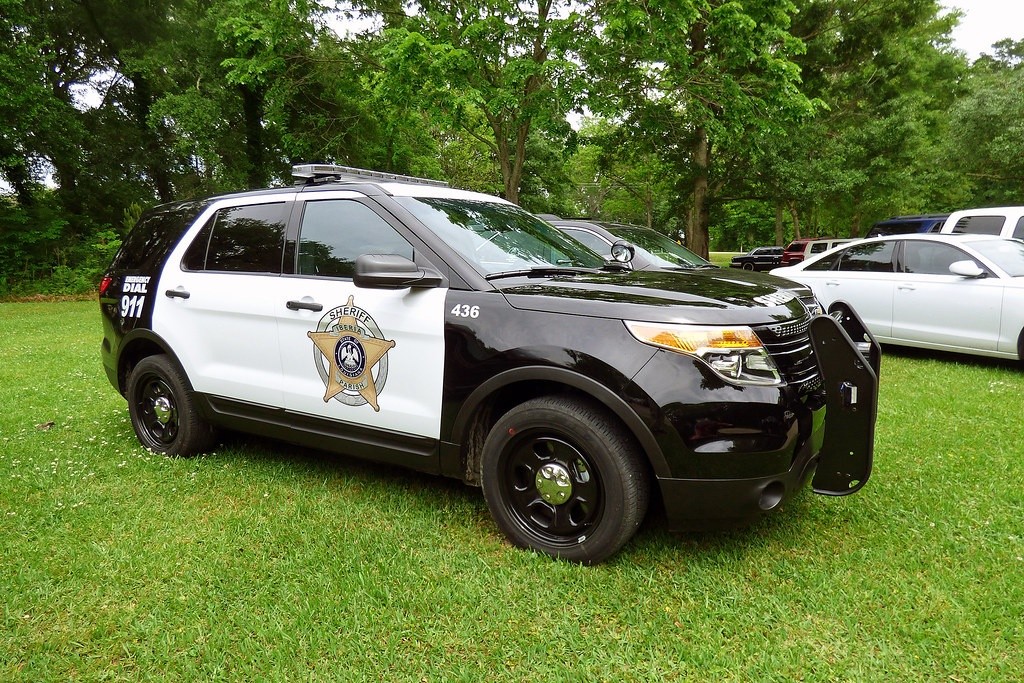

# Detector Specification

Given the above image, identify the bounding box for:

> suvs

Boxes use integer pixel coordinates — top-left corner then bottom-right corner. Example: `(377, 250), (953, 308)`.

(99, 165), (881, 566)
(865, 206), (1024, 239)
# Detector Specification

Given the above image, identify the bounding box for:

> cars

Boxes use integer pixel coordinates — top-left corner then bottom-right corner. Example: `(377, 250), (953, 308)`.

(730, 239), (859, 271)
(769, 234), (1024, 368)
